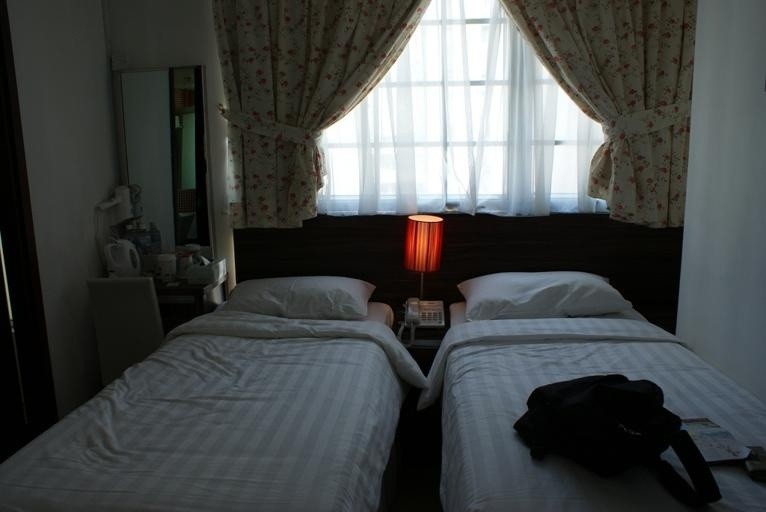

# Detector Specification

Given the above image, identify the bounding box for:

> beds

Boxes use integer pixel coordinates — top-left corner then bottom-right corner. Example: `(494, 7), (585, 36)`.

(1, 301), (396, 512)
(442, 296), (765, 512)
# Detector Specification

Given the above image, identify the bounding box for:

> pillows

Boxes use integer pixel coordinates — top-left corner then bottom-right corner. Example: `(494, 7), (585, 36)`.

(220, 272), (381, 318)
(455, 270), (631, 323)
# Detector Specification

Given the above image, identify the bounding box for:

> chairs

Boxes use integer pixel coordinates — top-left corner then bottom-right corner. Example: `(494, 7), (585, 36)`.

(86, 275), (168, 388)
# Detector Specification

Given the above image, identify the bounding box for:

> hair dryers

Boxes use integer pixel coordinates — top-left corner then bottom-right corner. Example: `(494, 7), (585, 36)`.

(96, 186), (131, 223)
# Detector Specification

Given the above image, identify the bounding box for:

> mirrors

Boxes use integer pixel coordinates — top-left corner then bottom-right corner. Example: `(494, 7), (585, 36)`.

(107, 64), (210, 256)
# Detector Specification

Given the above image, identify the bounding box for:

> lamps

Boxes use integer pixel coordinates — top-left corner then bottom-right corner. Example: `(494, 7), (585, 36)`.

(404, 213), (444, 298)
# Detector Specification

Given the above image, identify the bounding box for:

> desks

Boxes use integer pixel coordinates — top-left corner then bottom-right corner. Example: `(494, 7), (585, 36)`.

(100, 273), (230, 333)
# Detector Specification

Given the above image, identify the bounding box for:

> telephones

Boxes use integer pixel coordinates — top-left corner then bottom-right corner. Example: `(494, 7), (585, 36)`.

(404, 297), (445, 329)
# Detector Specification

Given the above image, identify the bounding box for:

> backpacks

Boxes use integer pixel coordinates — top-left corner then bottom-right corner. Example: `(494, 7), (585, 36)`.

(513, 374), (723, 507)
